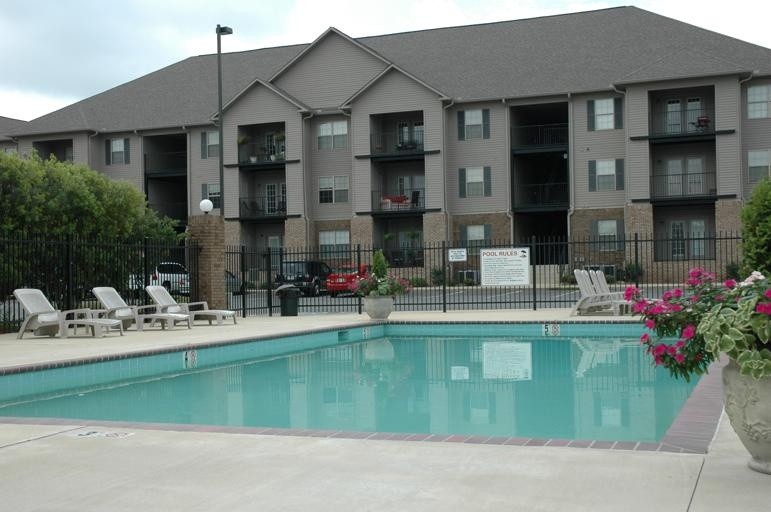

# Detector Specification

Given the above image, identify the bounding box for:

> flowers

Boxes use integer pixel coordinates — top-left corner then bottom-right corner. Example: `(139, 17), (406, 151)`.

(620, 268), (771, 380)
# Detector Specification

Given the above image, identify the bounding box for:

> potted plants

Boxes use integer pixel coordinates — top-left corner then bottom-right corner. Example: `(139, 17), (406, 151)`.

(347, 249), (411, 321)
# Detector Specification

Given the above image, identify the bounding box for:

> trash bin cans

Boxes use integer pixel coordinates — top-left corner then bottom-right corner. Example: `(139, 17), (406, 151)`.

(279, 286), (301, 316)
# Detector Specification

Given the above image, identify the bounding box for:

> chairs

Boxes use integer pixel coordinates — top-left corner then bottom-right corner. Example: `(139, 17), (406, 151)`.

(568, 268), (663, 316)
(12, 285), (238, 337)
(410, 191), (420, 208)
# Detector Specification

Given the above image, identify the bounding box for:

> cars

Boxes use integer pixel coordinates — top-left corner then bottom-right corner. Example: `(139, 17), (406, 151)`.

(274, 260), (333, 296)
(327, 263), (375, 298)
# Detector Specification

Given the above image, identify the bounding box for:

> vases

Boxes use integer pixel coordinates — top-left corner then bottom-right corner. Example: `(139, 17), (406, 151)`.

(721, 356), (771, 475)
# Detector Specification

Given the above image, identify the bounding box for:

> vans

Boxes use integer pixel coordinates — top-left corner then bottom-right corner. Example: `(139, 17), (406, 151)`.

(128, 261), (190, 295)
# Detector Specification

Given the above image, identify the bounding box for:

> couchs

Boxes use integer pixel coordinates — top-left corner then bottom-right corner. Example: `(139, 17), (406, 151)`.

(381, 194), (411, 211)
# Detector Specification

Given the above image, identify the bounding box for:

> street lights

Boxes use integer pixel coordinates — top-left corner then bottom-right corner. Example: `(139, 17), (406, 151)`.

(216, 24), (233, 216)
